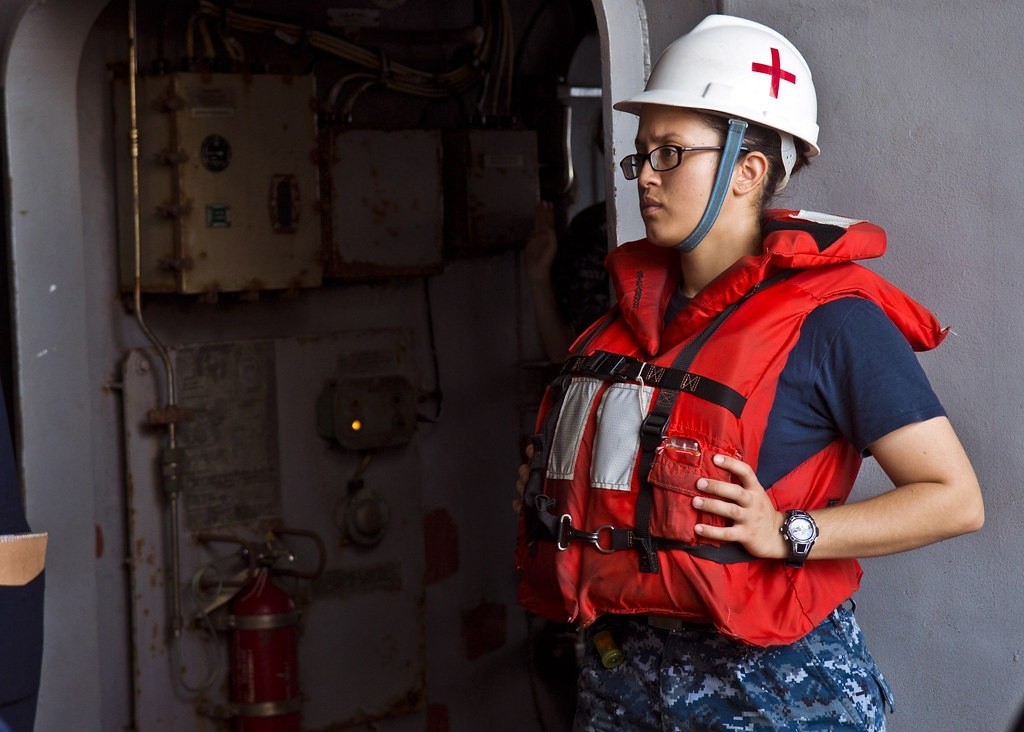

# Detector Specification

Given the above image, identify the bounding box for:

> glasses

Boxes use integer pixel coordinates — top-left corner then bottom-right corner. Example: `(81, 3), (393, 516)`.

(619, 144), (749, 180)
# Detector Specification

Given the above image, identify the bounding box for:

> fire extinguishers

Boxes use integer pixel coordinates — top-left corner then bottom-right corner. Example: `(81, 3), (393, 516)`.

(224, 545), (308, 732)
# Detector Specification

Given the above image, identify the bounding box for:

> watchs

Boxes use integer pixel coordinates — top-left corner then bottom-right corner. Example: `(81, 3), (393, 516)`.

(780, 509), (819, 568)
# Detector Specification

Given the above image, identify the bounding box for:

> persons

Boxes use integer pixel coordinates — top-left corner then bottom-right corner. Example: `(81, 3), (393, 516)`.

(513, 15), (985, 732)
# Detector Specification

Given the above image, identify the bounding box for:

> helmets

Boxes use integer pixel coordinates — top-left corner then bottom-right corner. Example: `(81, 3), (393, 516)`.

(613, 14), (821, 158)
